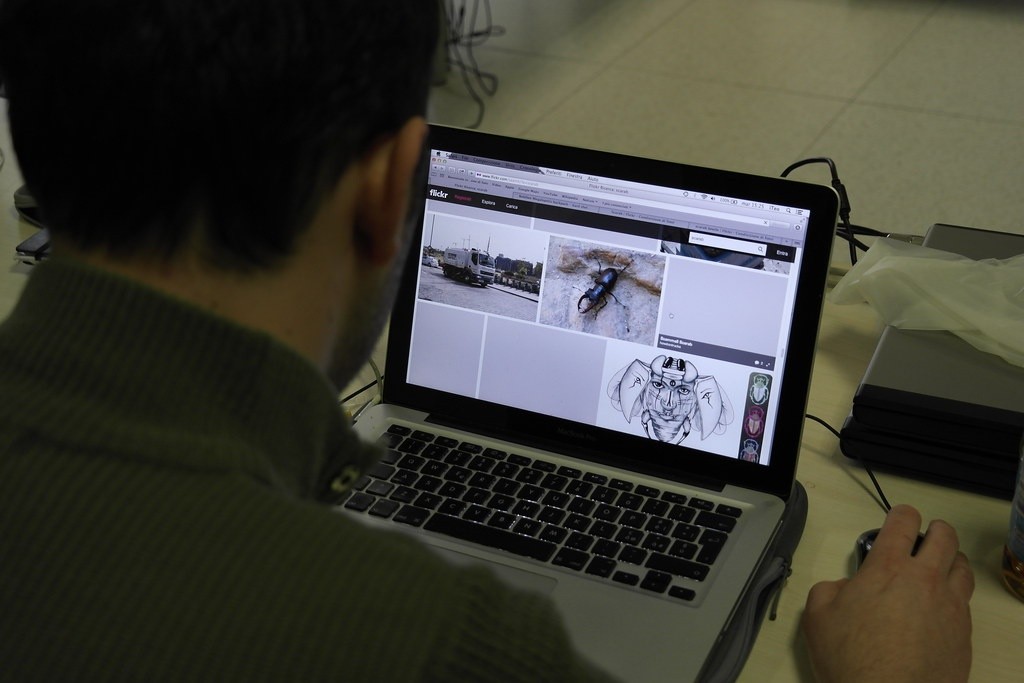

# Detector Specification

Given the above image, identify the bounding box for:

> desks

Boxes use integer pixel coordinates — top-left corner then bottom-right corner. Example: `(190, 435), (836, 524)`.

(0, 97), (1024, 683)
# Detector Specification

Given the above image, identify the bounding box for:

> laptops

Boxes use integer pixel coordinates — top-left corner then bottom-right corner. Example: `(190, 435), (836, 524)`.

(837, 224), (1024, 501)
(329, 124), (842, 683)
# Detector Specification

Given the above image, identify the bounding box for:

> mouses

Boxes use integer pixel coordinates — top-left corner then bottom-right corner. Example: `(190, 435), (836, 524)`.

(858, 528), (926, 568)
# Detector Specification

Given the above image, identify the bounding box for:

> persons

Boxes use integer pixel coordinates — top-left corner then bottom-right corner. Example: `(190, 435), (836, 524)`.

(0, 0), (976, 683)
